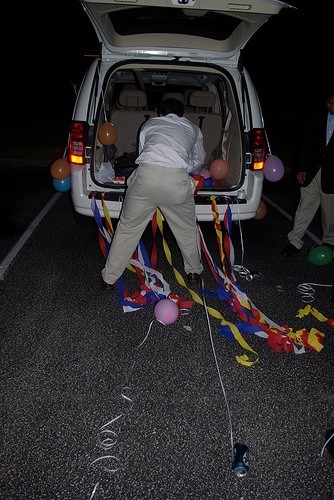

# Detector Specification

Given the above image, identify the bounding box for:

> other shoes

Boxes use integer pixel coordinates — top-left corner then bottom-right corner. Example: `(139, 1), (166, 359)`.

(281, 244), (300, 256)
(321, 243), (334, 251)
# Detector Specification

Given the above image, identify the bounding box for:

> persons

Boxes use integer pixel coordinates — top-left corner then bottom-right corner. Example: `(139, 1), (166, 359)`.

(268, 85), (333, 254)
(99, 97), (211, 288)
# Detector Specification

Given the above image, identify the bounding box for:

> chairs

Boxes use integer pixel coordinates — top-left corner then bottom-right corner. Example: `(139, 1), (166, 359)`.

(110, 84), (222, 164)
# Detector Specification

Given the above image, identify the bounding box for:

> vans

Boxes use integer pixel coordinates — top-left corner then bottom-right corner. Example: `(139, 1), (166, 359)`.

(61, 0), (298, 223)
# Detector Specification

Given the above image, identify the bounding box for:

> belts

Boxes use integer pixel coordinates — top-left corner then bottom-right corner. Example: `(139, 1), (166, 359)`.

(139, 162), (187, 173)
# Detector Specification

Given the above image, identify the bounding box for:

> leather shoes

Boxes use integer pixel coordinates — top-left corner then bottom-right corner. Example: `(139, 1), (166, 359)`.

(188, 273), (199, 284)
(99, 274), (113, 289)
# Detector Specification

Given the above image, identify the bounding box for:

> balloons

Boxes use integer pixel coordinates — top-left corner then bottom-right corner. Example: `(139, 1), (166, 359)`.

(51, 158), (71, 192)
(264, 152), (285, 188)
(250, 199), (267, 221)
(209, 158), (230, 181)
(154, 298), (179, 325)
(307, 242), (332, 266)
(98, 123), (116, 145)
